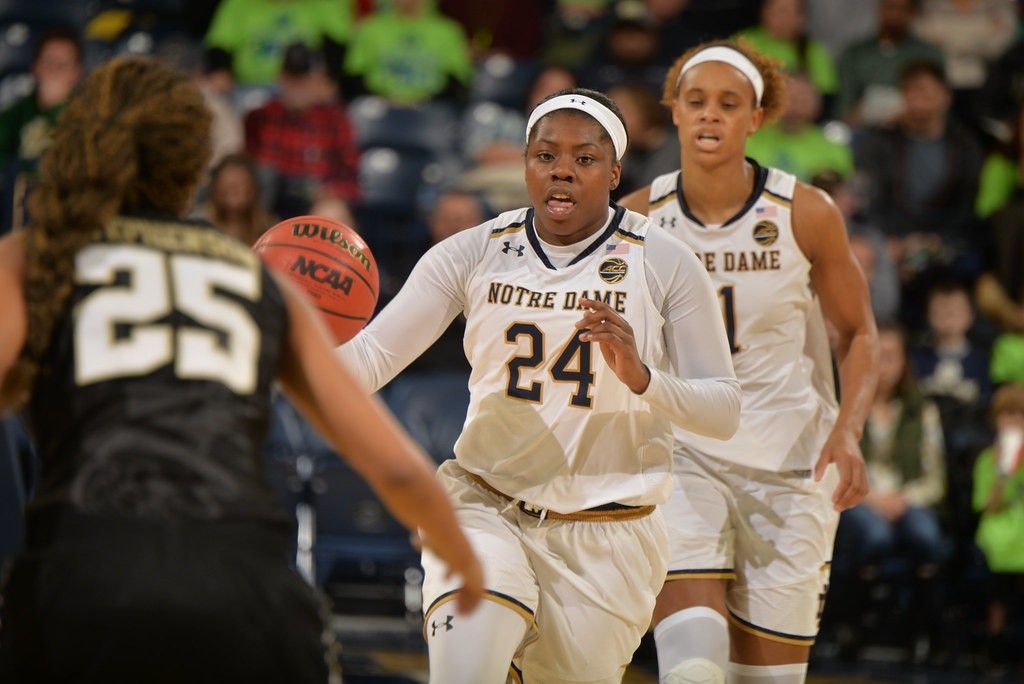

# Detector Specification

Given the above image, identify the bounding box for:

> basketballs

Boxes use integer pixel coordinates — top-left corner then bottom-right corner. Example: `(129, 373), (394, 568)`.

(250, 214), (381, 348)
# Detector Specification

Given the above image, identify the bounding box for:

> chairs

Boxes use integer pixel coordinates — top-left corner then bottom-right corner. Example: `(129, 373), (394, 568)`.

(0, 31), (1024, 683)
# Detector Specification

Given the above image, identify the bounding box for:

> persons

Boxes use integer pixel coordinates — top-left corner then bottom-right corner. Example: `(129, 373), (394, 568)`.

(334, 88), (742, 684)
(731, 0), (1024, 684)
(615, 41), (881, 684)
(1, 55), (482, 684)
(0, 0), (731, 376)
